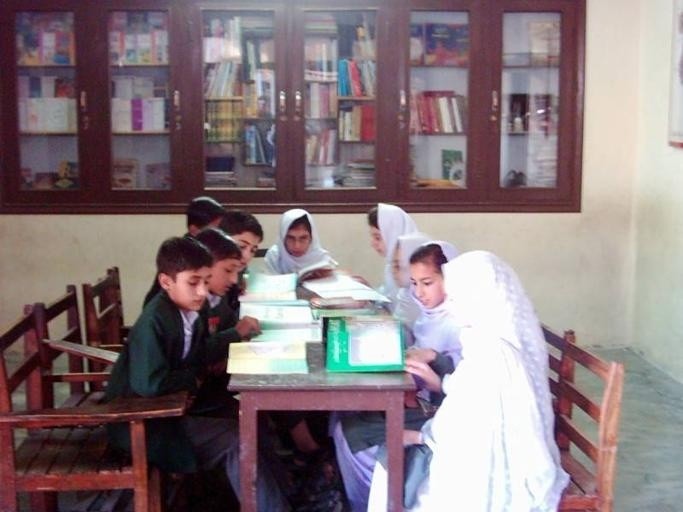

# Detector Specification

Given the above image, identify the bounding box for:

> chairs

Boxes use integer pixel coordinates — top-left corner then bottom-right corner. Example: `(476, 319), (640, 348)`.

(0, 266), (623, 512)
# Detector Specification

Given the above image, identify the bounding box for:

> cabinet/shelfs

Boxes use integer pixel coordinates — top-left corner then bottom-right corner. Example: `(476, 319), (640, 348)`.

(0, 0), (587, 214)
